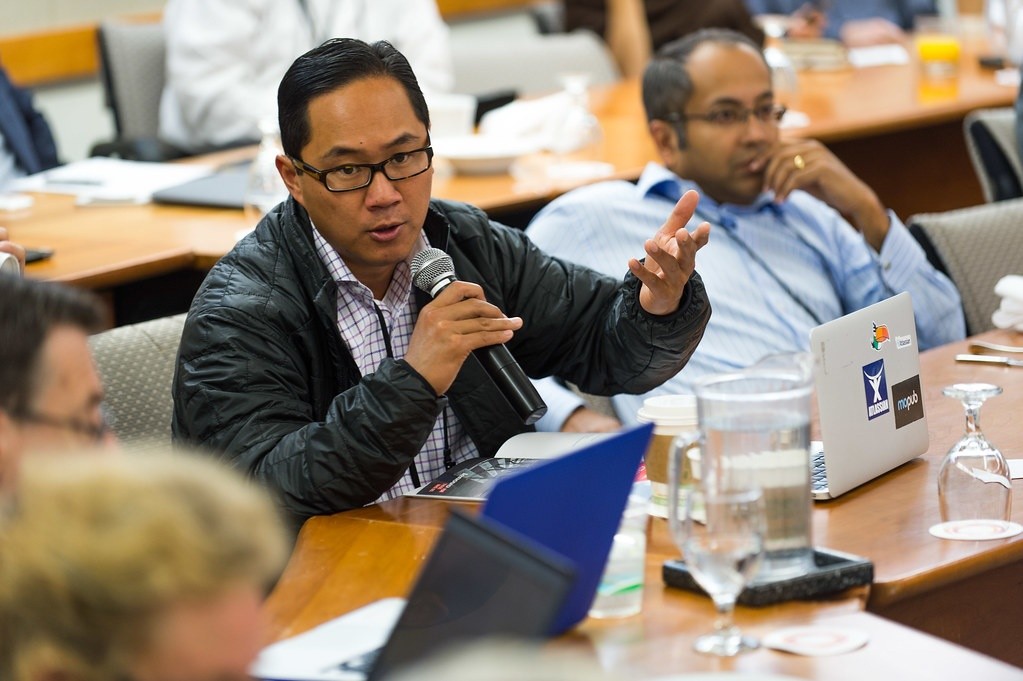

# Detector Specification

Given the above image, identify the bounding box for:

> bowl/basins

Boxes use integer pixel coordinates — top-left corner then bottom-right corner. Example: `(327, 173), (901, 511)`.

(427, 134), (541, 175)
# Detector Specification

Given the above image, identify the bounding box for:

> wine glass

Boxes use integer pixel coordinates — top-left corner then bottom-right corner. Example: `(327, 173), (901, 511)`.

(679, 481), (767, 656)
(936, 384), (1014, 536)
(241, 119), (289, 231)
(759, 24), (796, 117)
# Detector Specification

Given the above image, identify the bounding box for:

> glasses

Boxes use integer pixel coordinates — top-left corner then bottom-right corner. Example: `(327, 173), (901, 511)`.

(0, 399), (112, 445)
(286, 130), (434, 193)
(666, 106), (790, 128)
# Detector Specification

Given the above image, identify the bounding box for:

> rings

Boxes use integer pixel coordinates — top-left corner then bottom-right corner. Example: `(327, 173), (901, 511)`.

(794, 154), (805, 171)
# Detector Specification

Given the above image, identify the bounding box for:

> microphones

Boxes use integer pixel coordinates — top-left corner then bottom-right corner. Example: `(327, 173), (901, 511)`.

(410, 247), (548, 425)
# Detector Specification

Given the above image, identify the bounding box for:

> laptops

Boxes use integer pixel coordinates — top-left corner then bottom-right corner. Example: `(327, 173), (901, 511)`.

(247, 423), (660, 681)
(811, 291), (929, 502)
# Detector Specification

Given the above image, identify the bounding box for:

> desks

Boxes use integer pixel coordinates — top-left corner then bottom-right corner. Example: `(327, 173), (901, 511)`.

(1, 0), (1023, 681)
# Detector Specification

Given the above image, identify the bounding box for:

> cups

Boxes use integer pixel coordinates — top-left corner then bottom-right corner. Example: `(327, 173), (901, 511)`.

(692, 449), (810, 551)
(587, 495), (650, 618)
(635, 393), (703, 501)
(911, 17), (963, 100)
(667, 373), (812, 581)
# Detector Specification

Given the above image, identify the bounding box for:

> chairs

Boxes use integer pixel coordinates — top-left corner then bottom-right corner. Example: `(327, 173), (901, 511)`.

(88, 312), (186, 452)
(88, 20), (181, 163)
(907, 197), (1023, 338)
(456, 31), (623, 106)
(964, 106), (1023, 204)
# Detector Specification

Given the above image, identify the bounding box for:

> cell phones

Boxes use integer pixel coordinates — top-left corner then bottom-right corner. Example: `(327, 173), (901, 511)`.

(662, 549), (875, 606)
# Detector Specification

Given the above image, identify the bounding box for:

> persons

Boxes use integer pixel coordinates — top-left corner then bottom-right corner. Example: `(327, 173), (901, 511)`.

(562, 0), (939, 76)
(0, 441), (288, 681)
(520, 29), (963, 434)
(170, 38), (713, 518)
(159, 0), (450, 153)
(0, 272), (119, 489)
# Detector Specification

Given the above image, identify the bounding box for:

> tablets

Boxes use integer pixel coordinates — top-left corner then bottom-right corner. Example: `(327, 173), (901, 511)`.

(154, 170), (247, 208)
